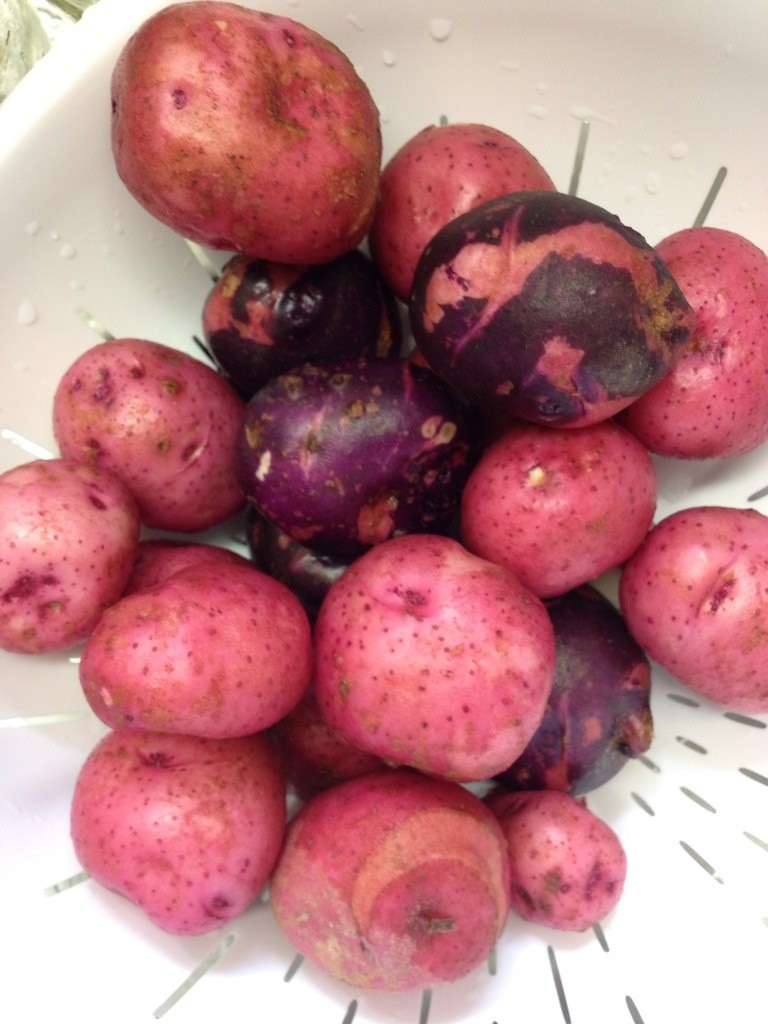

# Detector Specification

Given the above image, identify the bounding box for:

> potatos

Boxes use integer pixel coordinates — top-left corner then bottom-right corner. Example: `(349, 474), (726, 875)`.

(1, 0), (768, 991)
